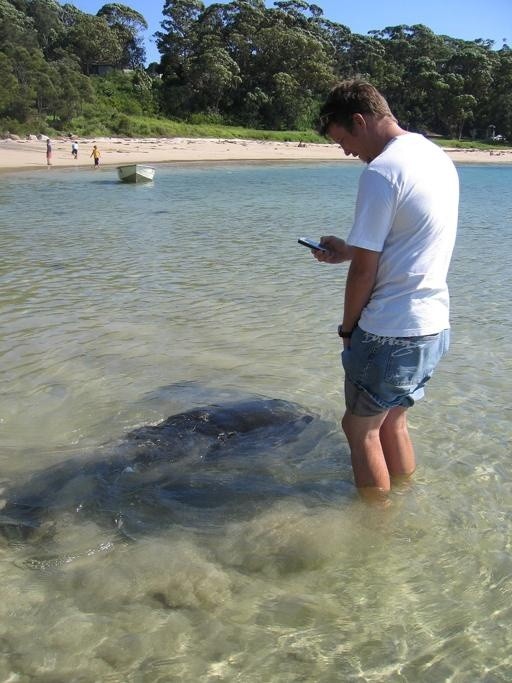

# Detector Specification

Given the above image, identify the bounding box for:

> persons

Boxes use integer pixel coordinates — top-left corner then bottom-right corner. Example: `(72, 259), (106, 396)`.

(47, 138), (53, 165)
(72, 140), (79, 159)
(309, 75), (460, 508)
(89, 144), (102, 168)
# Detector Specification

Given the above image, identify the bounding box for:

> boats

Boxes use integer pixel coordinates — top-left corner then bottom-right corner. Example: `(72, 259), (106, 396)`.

(116, 163), (155, 184)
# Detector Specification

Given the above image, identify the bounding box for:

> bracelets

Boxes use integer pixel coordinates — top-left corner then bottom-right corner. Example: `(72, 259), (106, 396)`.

(337, 324), (352, 339)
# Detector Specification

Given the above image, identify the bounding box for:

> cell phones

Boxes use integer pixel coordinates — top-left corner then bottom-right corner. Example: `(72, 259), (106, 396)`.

(298, 236), (329, 251)
